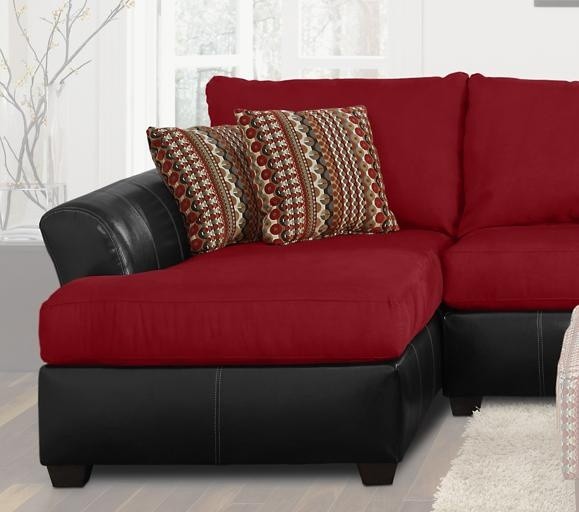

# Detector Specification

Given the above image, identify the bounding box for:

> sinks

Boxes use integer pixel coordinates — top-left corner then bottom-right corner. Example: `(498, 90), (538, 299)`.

(436, 73), (579, 418)
(39, 72), (466, 488)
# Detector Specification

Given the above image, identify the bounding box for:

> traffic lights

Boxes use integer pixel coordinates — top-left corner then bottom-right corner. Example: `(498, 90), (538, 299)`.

(460, 75), (579, 236)
(146, 122), (257, 251)
(204, 71), (469, 233)
(232, 106), (399, 245)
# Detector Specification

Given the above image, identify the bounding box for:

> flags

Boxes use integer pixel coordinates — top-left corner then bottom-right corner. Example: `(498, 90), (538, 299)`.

(0, 180), (71, 243)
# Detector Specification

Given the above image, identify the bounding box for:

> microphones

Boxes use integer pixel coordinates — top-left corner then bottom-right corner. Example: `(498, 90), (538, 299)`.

(555, 305), (579, 479)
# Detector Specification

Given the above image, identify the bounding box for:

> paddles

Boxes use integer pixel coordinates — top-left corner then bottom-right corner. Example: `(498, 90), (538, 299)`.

(431, 394), (579, 512)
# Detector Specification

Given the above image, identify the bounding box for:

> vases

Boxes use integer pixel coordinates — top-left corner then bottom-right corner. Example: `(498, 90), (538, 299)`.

(0, 0), (134, 231)
